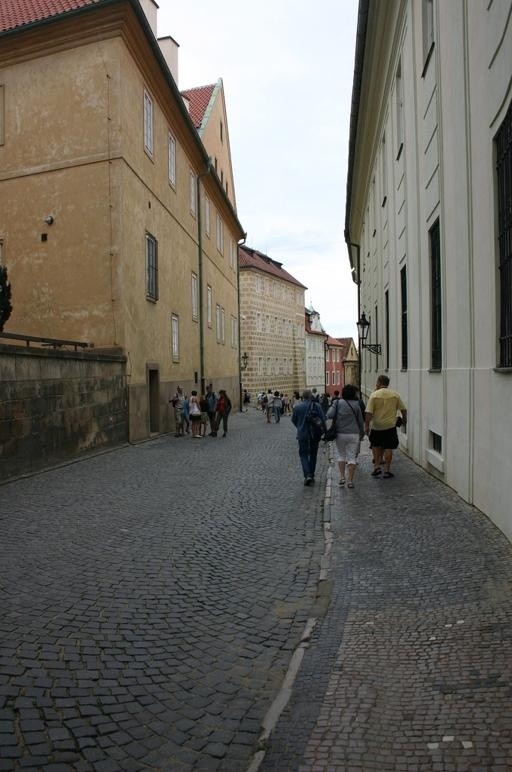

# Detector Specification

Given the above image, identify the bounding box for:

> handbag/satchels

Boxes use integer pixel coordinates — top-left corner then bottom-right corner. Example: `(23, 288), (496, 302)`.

(323, 421), (338, 442)
(396, 416), (403, 428)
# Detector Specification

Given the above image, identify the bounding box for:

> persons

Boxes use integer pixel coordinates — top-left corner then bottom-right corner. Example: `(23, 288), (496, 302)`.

(364, 375), (407, 479)
(327, 384), (365, 488)
(168, 385), (367, 439)
(291, 390), (327, 485)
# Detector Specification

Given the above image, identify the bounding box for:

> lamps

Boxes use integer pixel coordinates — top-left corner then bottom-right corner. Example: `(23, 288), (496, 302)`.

(354, 310), (382, 357)
(239, 352), (249, 370)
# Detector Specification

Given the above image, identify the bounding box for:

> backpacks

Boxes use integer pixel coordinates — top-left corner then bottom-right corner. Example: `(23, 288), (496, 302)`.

(306, 401), (326, 442)
(172, 393), (179, 408)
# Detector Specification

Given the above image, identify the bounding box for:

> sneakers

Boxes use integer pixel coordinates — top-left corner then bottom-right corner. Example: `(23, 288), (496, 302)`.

(371, 467), (382, 476)
(347, 480), (354, 489)
(191, 431), (227, 439)
(382, 472), (394, 478)
(338, 479), (346, 487)
(304, 474), (315, 487)
